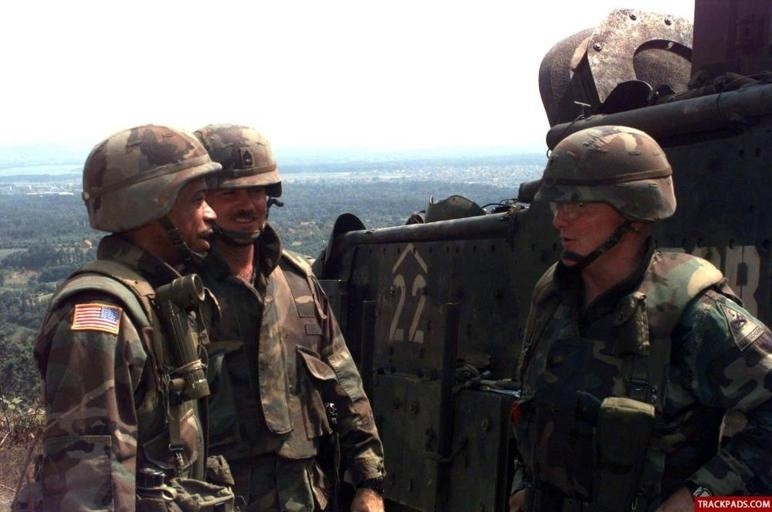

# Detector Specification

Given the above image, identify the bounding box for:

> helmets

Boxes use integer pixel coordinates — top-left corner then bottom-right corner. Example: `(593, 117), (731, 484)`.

(425, 194), (486, 225)
(82, 124), (224, 233)
(192, 122), (282, 197)
(533, 124), (678, 221)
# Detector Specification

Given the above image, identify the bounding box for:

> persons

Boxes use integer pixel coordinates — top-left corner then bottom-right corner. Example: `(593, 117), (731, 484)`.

(506, 125), (771, 512)
(32, 123), (226, 509)
(184, 123), (385, 512)
